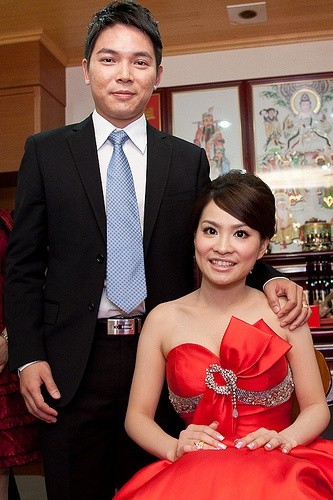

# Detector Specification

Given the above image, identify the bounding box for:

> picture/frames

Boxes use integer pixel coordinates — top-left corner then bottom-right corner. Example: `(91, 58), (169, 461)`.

(144, 71), (333, 260)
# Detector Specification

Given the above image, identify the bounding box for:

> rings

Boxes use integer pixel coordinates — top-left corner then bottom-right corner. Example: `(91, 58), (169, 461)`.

(194, 440), (203, 449)
(303, 304), (308, 308)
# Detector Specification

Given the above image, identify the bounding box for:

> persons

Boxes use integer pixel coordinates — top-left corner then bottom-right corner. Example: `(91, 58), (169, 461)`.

(124, 171), (333, 500)
(0, 209), (47, 500)
(4, 0), (312, 500)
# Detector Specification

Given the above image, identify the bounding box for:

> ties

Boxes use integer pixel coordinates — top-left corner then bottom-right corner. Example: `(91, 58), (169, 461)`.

(105, 129), (147, 313)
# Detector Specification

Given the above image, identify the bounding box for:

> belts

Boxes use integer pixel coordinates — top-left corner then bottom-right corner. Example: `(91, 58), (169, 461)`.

(95, 314), (147, 338)
